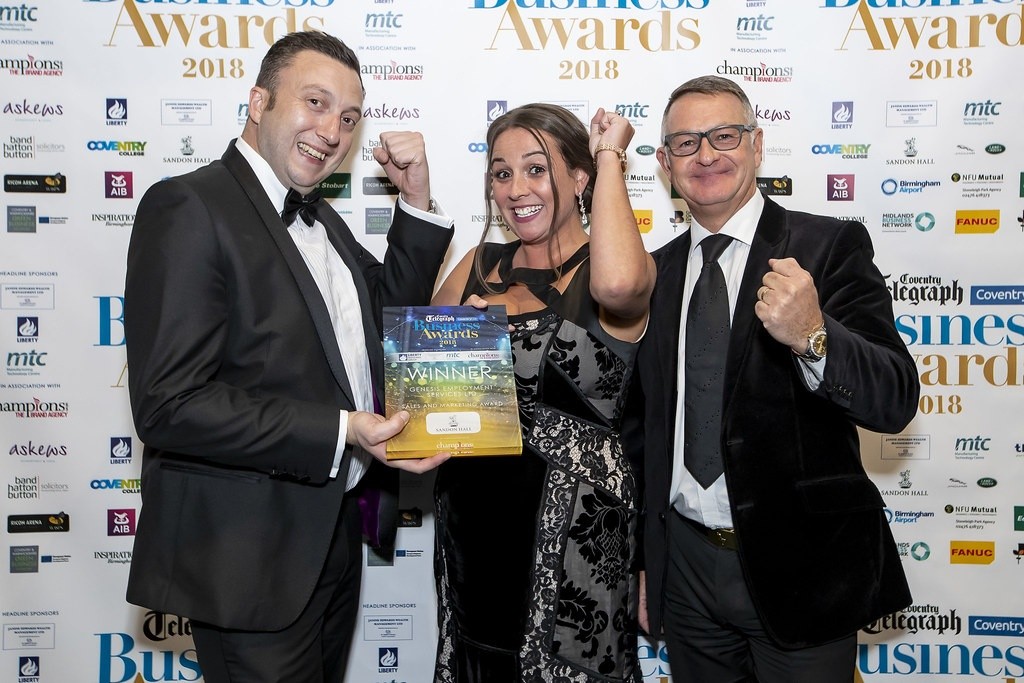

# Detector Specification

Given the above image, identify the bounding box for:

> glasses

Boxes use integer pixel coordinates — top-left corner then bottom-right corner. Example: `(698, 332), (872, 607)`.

(662, 125), (752, 156)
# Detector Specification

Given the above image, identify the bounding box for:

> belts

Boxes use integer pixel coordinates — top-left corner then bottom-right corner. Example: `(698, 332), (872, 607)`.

(678, 513), (735, 550)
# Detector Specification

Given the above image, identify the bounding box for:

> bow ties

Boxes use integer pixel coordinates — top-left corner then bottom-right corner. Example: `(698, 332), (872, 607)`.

(282, 187), (321, 228)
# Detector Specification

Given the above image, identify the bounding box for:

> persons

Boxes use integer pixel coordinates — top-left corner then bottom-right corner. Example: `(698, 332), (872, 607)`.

(125, 31), (455, 683)
(625, 75), (920, 683)
(430, 103), (656, 683)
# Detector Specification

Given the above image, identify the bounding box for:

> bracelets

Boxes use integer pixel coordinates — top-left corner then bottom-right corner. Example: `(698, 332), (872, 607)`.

(429, 200), (437, 214)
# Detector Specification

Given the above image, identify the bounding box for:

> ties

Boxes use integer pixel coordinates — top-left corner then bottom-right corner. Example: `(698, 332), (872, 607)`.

(683, 234), (735, 490)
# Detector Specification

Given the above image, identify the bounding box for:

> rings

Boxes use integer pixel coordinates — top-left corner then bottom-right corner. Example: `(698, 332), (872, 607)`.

(761, 288), (769, 300)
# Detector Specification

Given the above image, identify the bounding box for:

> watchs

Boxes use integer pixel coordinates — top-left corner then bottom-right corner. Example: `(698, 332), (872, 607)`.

(792, 321), (827, 361)
(593, 144), (627, 172)
